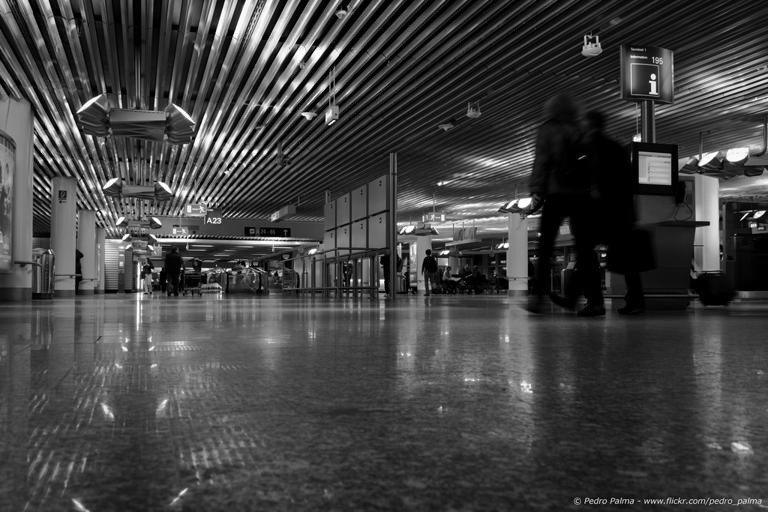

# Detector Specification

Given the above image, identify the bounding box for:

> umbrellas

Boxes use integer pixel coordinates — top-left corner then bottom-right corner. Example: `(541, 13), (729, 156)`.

(550, 293), (575, 310)
(618, 304), (647, 315)
(576, 305), (605, 317)
(525, 295), (544, 314)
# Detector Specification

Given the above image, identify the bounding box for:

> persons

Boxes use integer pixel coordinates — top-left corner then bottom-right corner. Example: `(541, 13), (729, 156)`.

(421, 248), (438, 297)
(163, 246), (183, 296)
(380, 253), (391, 298)
(438, 263), (496, 296)
(75, 249), (84, 296)
(142, 257), (155, 295)
(159, 267), (167, 294)
(527, 258), (535, 296)
(226, 269), (280, 288)
(549, 108), (648, 317)
(517, 91), (608, 318)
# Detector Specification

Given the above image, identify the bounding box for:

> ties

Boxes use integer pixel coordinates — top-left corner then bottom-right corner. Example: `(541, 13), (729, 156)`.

(630, 142), (678, 196)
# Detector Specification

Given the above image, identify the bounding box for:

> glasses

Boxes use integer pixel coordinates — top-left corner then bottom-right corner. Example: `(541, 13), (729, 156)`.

(100, 151), (174, 256)
(678, 114), (768, 179)
(466, 101), (481, 119)
(581, 31), (603, 57)
(496, 184), (544, 217)
(394, 194), (440, 237)
(74, 50), (196, 147)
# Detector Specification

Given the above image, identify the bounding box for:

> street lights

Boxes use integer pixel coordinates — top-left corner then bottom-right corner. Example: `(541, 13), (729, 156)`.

(608, 228), (657, 275)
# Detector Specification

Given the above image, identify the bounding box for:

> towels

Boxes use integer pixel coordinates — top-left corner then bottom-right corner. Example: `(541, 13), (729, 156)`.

(397, 272), (407, 293)
(31, 248), (56, 299)
